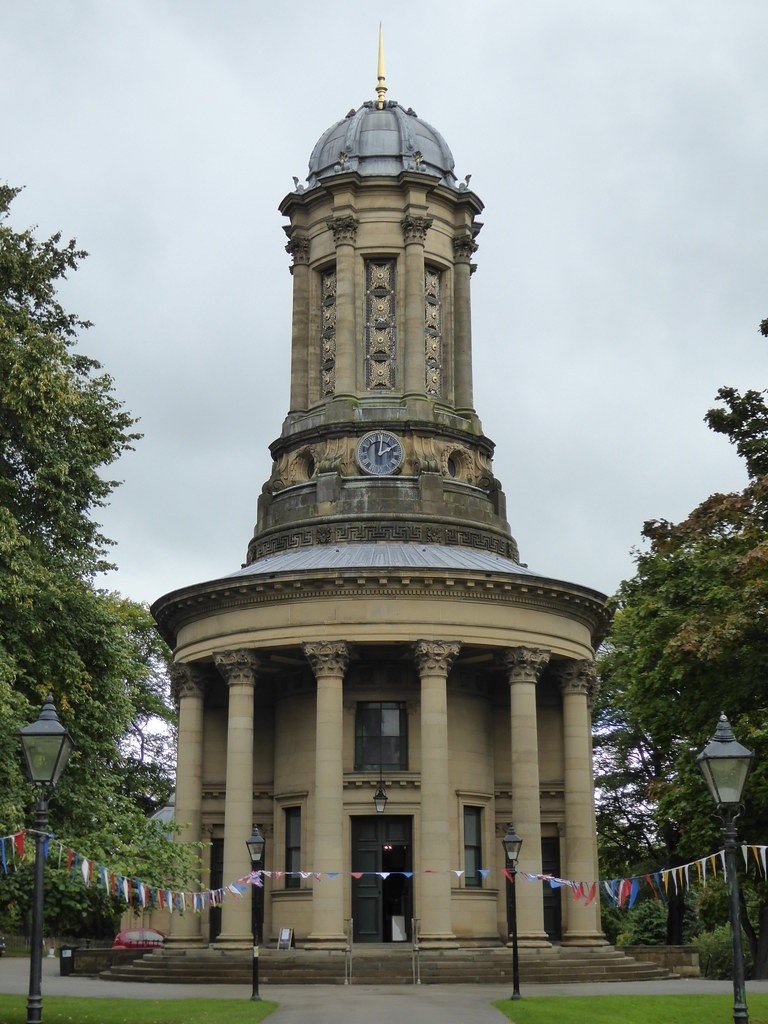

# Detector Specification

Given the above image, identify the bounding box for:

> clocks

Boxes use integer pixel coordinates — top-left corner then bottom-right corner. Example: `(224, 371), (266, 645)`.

(354, 429), (406, 475)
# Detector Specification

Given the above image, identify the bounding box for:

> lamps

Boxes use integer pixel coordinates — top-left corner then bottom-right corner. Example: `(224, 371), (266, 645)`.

(373, 772), (389, 813)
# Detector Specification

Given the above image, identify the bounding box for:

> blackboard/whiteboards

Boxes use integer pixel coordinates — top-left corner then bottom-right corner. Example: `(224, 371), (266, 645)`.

(278, 927), (295, 942)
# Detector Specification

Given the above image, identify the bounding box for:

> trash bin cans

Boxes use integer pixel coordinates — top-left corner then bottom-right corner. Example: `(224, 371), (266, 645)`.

(58, 945), (74, 976)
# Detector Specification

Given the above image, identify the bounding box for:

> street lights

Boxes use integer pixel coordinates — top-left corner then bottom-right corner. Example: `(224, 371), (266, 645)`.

(8, 691), (79, 1023)
(696, 709), (755, 1024)
(500, 820), (525, 1001)
(243, 822), (265, 1002)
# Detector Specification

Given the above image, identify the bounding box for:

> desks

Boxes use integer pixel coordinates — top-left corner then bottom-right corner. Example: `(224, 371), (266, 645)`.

(391, 915), (407, 941)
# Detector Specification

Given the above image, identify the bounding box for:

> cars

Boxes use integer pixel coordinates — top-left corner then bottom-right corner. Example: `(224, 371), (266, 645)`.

(111, 926), (166, 949)
